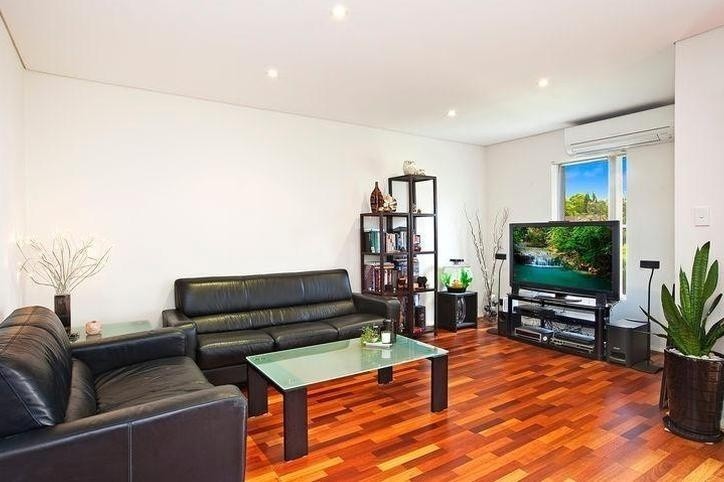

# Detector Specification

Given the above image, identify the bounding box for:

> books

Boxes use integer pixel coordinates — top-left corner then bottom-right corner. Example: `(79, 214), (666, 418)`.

(364, 226), (421, 294)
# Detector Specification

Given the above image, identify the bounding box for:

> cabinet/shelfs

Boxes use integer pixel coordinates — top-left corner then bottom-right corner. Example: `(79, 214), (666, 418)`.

(359, 172), (440, 340)
(487, 293), (609, 359)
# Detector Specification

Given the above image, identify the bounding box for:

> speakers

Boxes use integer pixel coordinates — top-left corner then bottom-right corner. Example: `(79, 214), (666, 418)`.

(606, 319), (650, 367)
(499, 311), (520, 337)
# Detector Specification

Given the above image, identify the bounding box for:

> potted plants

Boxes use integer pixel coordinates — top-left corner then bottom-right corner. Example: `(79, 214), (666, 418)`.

(360, 324), (381, 346)
(631, 239), (724, 444)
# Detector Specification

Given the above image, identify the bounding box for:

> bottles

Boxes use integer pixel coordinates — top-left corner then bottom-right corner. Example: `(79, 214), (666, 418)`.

(379, 319), (395, 344)
(370, 181), (384, 214)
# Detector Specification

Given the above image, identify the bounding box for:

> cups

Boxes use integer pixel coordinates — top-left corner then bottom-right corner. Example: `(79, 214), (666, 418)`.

(84, 320), (104, 336)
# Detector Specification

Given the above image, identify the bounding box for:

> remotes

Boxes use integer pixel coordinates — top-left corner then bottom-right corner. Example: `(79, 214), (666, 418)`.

(365, 342), (393, 348)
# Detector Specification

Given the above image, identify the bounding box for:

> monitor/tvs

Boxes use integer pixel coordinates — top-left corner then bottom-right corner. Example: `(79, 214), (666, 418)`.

(509, 219), (621, 302)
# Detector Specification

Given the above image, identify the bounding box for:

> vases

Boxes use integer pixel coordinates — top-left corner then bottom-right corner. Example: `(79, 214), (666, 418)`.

(53, 294), (71, 335)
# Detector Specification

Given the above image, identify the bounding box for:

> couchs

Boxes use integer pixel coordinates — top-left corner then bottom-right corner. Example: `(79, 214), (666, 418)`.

(160, 269), (401, 396)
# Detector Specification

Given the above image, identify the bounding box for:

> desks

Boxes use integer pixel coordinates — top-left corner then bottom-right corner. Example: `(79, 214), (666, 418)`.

(437, 288), (479, 333)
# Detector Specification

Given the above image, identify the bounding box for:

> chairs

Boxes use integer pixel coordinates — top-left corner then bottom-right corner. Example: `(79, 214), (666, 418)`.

(0, 306), (245, 482)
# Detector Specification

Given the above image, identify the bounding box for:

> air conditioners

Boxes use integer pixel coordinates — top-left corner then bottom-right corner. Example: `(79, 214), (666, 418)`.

(563, 103), (675, 157)
